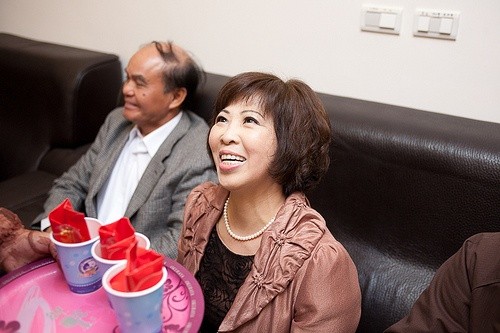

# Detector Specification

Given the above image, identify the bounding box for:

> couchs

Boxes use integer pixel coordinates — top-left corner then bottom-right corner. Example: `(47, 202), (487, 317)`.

(0, 31), (500, 333)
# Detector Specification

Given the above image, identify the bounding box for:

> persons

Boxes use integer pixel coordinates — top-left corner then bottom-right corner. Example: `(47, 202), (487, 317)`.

(29, 39), (220, 261)
(380, 232), (500, 333)
(0, 207), (62, 273)
(176, 72), (361, 333)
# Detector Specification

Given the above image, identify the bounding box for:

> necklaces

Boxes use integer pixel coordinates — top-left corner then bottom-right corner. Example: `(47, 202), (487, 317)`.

(224, 196), (278, 240)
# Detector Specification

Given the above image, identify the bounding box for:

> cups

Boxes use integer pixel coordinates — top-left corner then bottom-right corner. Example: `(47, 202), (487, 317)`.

(101, 260), (167, 333)
(49, 217), (106, 294)
(91, 232), (151, 276)
